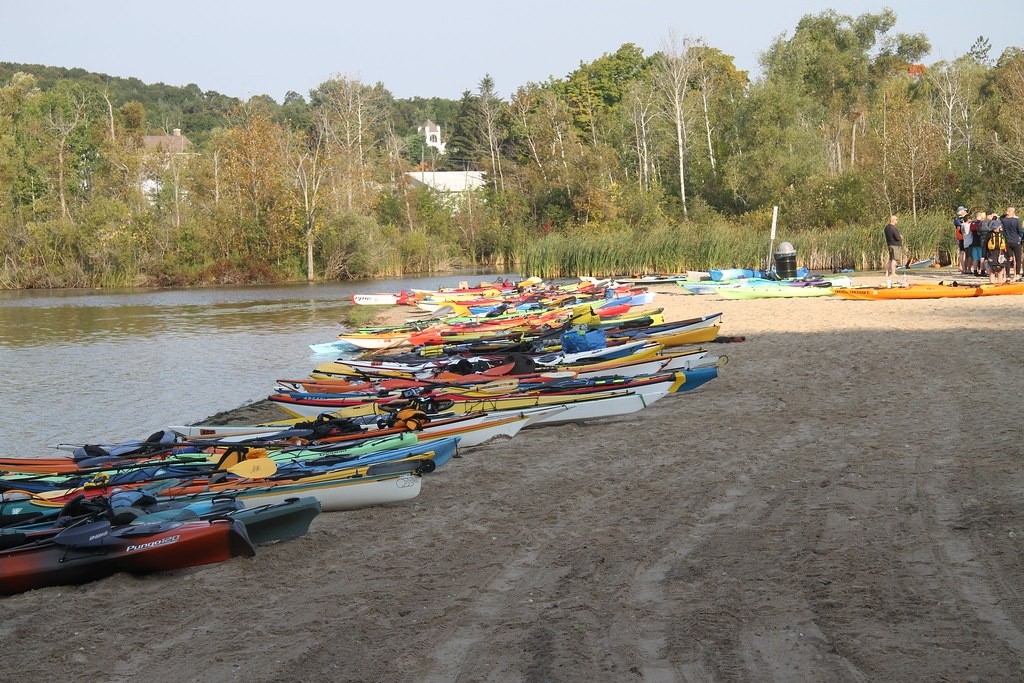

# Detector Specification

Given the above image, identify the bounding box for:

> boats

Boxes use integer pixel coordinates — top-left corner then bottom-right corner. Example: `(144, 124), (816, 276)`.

(0, 267), (1024, 598)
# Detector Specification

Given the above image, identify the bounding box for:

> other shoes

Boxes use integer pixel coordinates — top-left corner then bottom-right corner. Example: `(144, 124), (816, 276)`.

(974, 274), (989, 277)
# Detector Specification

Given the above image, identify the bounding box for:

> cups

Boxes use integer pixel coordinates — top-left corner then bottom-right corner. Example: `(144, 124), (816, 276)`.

(886, 280), (892, 288)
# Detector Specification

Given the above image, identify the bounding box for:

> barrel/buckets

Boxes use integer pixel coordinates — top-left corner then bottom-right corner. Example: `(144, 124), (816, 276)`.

(774, 253), (796, 280)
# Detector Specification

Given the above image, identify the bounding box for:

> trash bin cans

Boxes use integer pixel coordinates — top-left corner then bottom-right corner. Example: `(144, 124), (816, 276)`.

(774, 250), (796, 280)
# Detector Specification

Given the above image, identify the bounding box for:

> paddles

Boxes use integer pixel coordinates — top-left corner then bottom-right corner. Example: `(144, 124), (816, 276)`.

(3, 510), (61, 529)
(0, 427), (332, 481)
(420, 305), (593, 358)
(951, 281), (997, 287)
(33, 455), (279, 500)
(275, 363), (575, 398)
(4, 520), (112, 551)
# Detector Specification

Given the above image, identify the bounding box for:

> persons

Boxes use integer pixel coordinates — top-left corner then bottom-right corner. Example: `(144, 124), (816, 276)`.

(884, 216), (905, 289)
(954, 205), (1024, 285)
(504, 278), (510, 287)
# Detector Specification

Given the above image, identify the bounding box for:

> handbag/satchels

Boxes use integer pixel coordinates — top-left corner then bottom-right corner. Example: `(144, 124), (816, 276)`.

(560, 324), (607, 353)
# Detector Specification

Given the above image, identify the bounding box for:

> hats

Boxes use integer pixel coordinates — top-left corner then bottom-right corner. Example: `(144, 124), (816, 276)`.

(957, 206), (967, 212)
(990, 221), (1001, 229)
